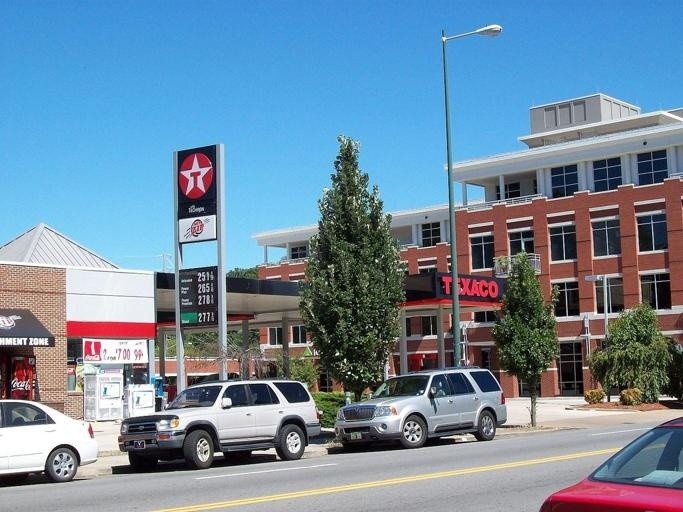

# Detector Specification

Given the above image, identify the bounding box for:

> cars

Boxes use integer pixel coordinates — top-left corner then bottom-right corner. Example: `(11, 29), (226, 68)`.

(539, 416), (683, 512)
(0, 399), (98, 483)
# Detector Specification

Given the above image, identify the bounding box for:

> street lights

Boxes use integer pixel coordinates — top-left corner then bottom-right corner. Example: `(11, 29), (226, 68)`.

(440, 23), (504, 369)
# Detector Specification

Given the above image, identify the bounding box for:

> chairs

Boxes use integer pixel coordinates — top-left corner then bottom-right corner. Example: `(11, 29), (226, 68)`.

(11, 413), (52, 427)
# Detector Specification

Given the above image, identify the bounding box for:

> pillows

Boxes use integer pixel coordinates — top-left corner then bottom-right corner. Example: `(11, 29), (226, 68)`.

(634, 470), (683, 485)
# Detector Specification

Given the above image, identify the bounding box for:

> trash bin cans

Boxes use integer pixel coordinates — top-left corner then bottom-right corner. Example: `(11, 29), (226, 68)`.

(155, 398), (162, 412)
(163, 386), (177, 404)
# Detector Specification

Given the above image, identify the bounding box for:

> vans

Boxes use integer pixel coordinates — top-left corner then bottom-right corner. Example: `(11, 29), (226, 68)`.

(335, 369), (508, 449)
(118, 380), (323, 470)
